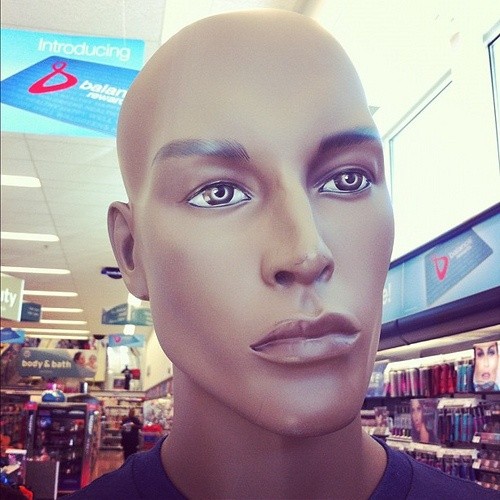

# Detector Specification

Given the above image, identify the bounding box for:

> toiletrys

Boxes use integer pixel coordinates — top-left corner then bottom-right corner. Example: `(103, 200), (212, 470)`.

(474, 397), (499, 490)
(394, 446), (473, 482)
(365, 357), (474, 398)
(361, 400), (479, 449)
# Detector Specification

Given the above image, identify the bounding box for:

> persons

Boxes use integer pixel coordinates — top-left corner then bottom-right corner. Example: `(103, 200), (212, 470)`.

(121, 409), (142, 461)
(411, 399), (436, 443)
(72, 352), (96, 369)
(473, 342), (498, 391)
(69, 11), (499, 500)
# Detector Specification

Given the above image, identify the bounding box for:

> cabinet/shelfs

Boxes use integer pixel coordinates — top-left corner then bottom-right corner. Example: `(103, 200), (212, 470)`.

(32, 403), (94, 493)
(89, 388), (146, 450)
(360, 203), (500, 500)
